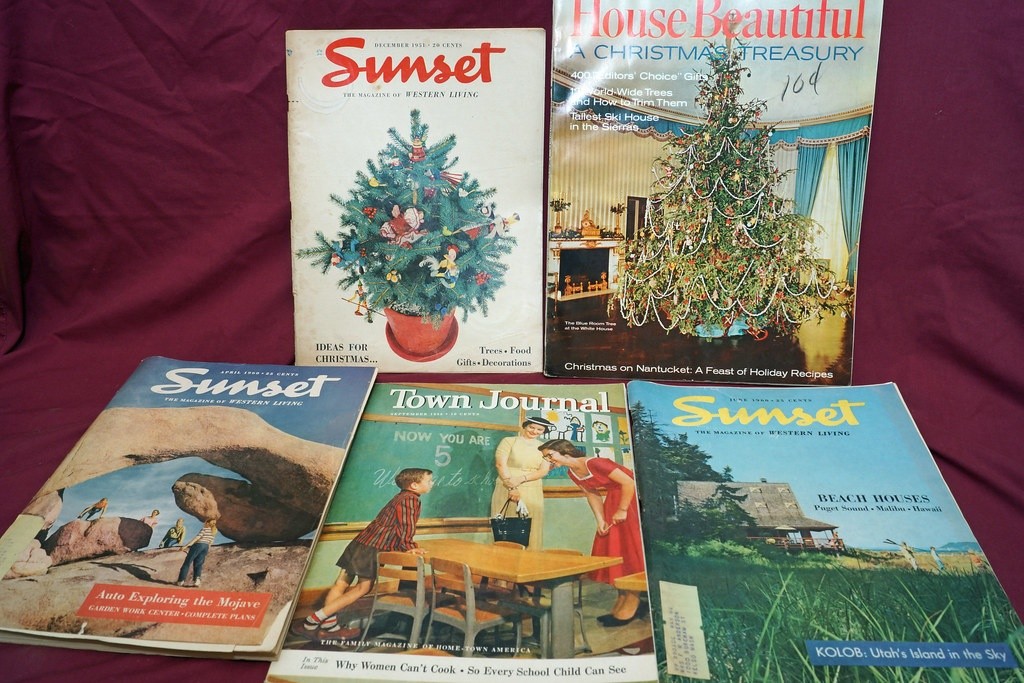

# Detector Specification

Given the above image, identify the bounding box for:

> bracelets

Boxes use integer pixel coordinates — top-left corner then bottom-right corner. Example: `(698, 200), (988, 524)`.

(618, 506), (628, 512)
(508, 486), (518, 490)
(523, 474), (528, 483)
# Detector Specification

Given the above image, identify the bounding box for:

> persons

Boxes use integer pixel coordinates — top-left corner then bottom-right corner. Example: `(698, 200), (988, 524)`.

(538, 439), (647, 626)
(827, 531), (844, 552)
(139, 510), (159, 529)
(967, 549), (989, 571)
(930, 547), (948, 572)
(289, 468), (434, 642)
(176, 518), (217, 587)
(900, 541), (919, 571)
(78, 497), (108, 522)
(158, 518), (186, 547)
(489, 419), (551, 549)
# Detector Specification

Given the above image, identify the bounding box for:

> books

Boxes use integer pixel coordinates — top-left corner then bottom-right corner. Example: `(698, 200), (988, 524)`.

(284, 27), (542, 375)
(623, 379), (1024, 683)
(542, 0), (886, 385)
(262, 383), (660, 683)
(3, 355), (382, 662)
